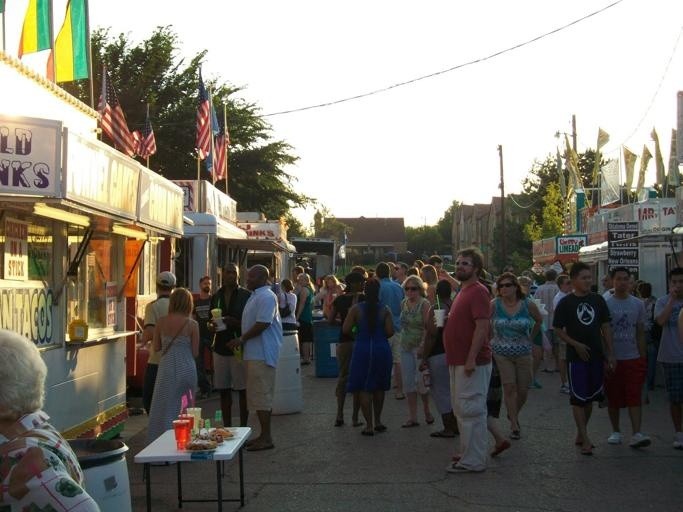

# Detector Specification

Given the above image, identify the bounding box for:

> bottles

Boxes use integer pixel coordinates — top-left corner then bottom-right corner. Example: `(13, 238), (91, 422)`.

(215, 410), (224, 430)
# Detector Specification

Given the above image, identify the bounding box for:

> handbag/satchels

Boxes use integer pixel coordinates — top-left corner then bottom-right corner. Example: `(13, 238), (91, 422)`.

(279, 304), (290, 317)
(542, 333), (553, 351)
(414, 370), (432, 394)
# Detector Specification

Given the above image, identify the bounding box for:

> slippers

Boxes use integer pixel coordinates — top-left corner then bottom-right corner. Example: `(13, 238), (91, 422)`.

(507, 415), (520, 439)
(244, 438), (273, 450)
(334, 414), (460, 437)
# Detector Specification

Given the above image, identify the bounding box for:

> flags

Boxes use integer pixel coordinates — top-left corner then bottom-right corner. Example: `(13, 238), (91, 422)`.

(208, 131), (229, 184)
(97, 70), (136, 159)
(194, 76), (210, 161)
(44, 1), (88, 83)
(205, 103), (221, 176)
(556, 124), (682, 201)
(16, 1), (52, 61)
(134, 117), (157, 160)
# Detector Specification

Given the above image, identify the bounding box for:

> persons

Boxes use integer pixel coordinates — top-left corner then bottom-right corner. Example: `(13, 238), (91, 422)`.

(0, 328), (102, 512)
(140, 247), (683, 475)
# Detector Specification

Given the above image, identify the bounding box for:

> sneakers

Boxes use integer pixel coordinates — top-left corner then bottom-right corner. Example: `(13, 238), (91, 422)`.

(576, 432), (683, 454)
(447, 439), (511, 472)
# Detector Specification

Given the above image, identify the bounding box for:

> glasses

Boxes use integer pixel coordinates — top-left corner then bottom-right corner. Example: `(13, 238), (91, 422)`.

(499, 283), (513, 288)
(405, 287), (419, 290)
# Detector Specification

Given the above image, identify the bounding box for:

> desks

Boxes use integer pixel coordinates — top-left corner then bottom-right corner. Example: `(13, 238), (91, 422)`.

(133, 426), (252, 511)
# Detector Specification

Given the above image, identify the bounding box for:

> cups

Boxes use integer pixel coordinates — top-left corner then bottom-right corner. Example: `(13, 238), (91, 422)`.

(211, 308), (224, 331)
(533, 299), (546, 313)
(172, 407), (202, 449)
(433, 309), (447, 328)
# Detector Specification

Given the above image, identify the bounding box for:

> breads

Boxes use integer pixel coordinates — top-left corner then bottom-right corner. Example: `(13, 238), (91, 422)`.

(185, 428), (233, 450)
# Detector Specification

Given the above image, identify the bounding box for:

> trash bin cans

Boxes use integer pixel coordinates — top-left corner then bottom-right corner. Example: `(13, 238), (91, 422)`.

(313, 320), (342, 378)
(65, 439), (132, 512)
(270, 330), (304, 416)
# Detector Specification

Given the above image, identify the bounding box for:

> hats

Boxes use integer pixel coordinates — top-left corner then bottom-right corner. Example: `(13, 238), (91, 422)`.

(157, 271), (176, 287)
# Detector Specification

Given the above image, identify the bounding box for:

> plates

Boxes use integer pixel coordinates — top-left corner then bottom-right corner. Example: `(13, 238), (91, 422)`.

(187, 432), (236, 453)
